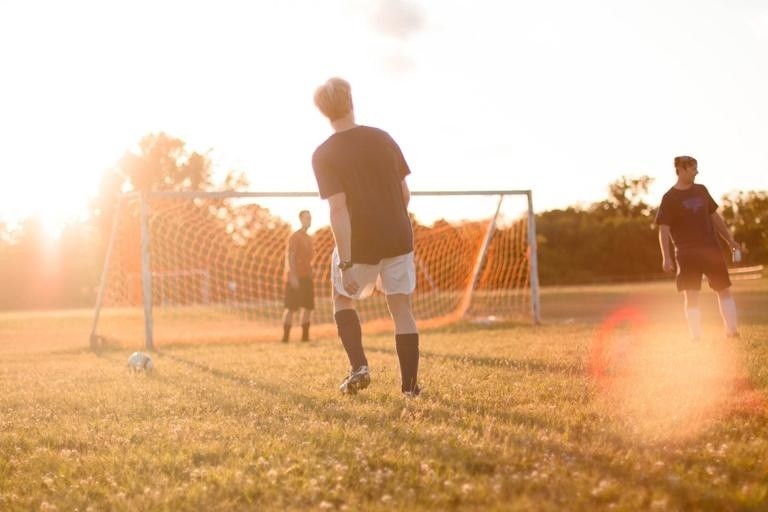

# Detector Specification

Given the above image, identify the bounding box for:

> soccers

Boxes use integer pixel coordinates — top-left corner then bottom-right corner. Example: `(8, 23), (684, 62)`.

(128, 352), (153, 373)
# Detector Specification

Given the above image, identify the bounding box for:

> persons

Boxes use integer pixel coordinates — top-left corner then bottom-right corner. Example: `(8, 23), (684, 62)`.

(281, 210), (314, 342)
(311, 79), (419, 396)
(655, 156), (740, 348)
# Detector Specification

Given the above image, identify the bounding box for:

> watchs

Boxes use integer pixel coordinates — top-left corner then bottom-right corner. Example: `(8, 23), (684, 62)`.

(338, 261), (353, 271)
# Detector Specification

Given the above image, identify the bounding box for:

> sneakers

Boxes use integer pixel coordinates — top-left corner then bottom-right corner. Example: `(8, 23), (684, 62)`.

(339, 365), (370, 395)
